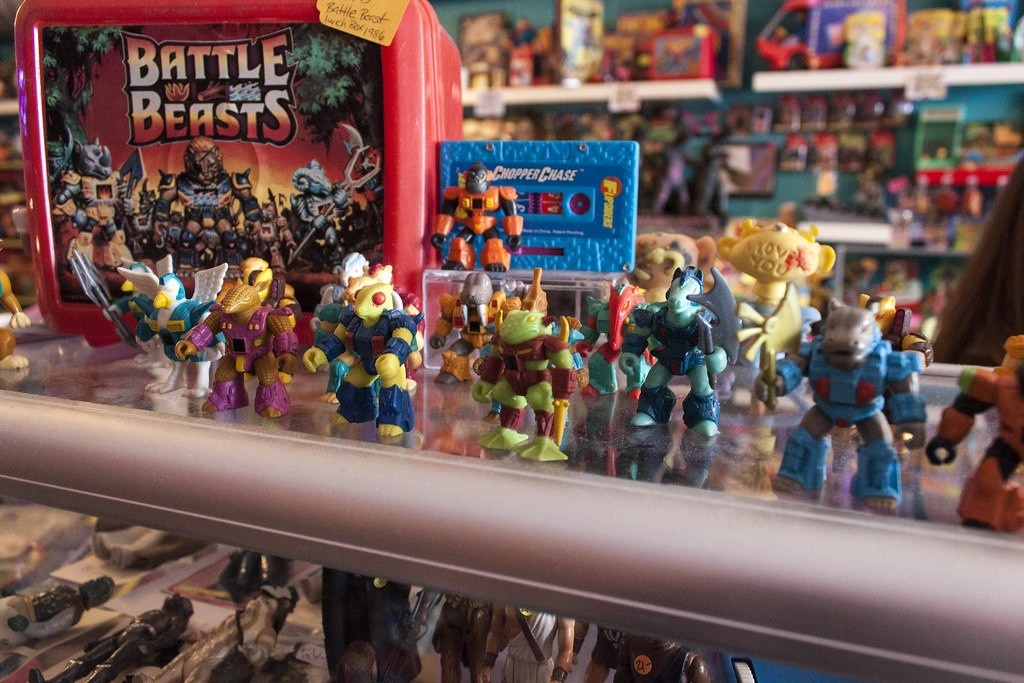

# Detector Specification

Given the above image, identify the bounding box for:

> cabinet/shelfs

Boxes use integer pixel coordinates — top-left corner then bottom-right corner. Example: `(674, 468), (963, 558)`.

(459, 66), (1023, 252)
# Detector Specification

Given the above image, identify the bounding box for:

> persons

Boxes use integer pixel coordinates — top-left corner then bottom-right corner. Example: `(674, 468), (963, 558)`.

(0, 575), (114, 648)
(89, 525), (712, 683)
(131, 580), (300, 683)
(28, 592), (194, 683)
